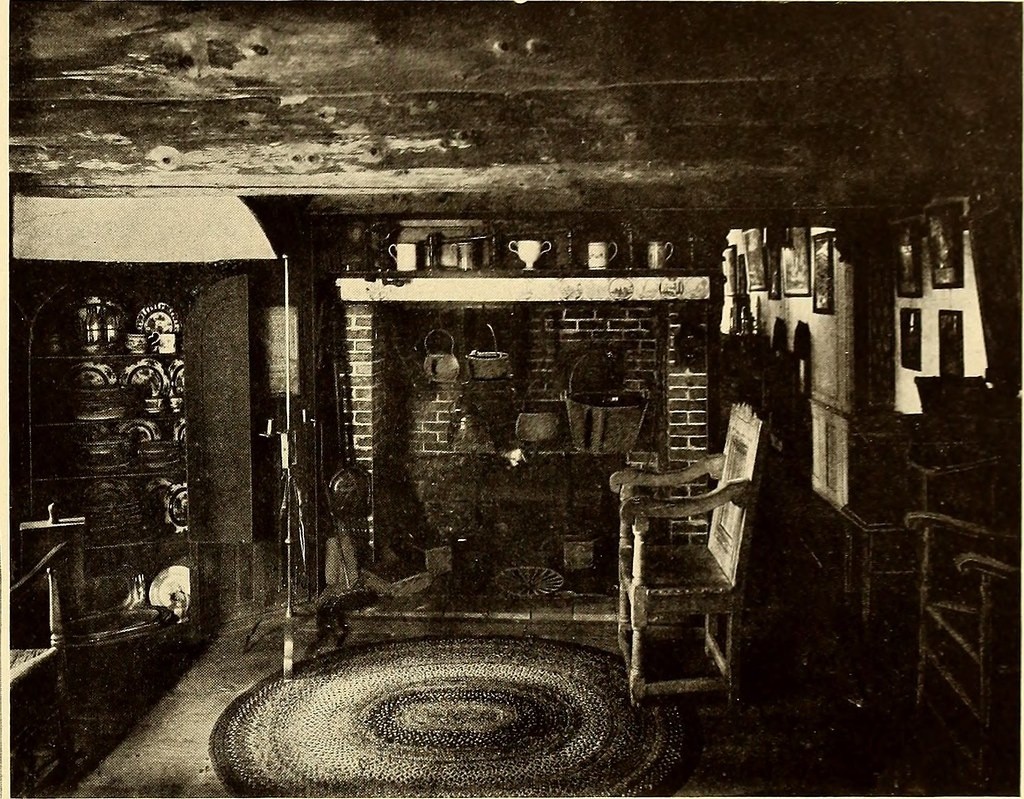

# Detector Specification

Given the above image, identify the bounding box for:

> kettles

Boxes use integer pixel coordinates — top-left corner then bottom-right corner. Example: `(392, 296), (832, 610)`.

(447, 405), (489, 448)
(511, 378), (559, 443)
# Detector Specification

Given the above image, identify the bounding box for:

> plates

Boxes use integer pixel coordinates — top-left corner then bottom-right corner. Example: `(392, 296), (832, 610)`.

(148, 566), (191, 624)
(71, 296), (188, 540)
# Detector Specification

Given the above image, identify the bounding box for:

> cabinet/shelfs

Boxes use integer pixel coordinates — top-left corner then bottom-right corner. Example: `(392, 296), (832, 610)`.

(25, 270), (257, 554)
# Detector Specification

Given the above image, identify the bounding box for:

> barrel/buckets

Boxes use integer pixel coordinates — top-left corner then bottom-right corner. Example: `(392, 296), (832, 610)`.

(423, 329), (460, 384)
(560, 345), (653, 454)
(465, 323), (510, 381)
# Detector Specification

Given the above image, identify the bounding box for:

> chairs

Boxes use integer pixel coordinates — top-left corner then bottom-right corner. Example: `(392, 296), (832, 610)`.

(903, 510), (1020, 797)
(10, 542), (77, 778)
(608, 400), (771, 709)
(901, 446), (1006, 525)
(839, 497), (917, 626)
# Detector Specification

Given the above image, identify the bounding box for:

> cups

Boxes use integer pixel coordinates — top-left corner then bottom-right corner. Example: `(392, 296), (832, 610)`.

(508, 240), (552, 271)
(389, 243), (417, 271)
(451, 242), (476, 271)
(144, 397), (165, 414)
(587, 241), (618, 271)
(646, 241), (674, 270)
(159, 334), (176, 354)
(170, 398), (183, 413)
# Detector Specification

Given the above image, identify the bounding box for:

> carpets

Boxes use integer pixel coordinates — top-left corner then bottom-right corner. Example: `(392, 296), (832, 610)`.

(207, 634), (704, 798)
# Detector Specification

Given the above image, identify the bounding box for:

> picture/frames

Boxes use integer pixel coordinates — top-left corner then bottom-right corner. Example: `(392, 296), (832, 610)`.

(812, 231), (836, 315)
(936, 308), (966, 378)
(924, 200), (964, 289)
(781, 227), (812, 298)
(723, 244), (737, 297)
(741, 228), (768, 292)
(898, 308), (922, 373)
(891, 219), (923, 298)
(762, 244), (782, 301)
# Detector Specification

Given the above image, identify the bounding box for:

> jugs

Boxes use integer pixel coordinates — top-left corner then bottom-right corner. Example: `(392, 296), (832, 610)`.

(125, 332), (160, 354)
